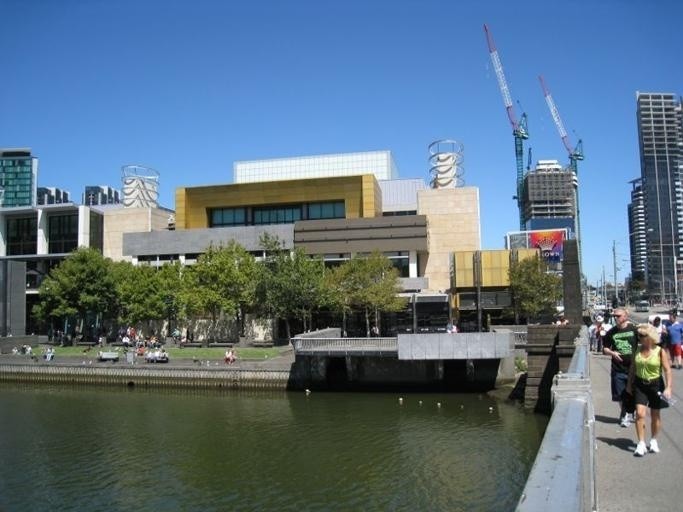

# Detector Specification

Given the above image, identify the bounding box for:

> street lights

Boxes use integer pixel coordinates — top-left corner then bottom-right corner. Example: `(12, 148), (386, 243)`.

(612, 228), (655, 299)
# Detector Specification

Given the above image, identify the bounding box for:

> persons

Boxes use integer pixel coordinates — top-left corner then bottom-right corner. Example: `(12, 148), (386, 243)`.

(587, 313), (683, 369)
(11, 325), (239, 370)
(602, 308), (638, 428)
(627, 323), (673, 456)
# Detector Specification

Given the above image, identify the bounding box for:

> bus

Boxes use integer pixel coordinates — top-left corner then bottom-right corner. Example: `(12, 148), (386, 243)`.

(635, 300), (649, 312)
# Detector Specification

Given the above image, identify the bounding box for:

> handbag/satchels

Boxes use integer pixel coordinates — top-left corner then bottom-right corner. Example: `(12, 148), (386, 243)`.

(621, 387), (635, 413)
(661, 332), (669, 343)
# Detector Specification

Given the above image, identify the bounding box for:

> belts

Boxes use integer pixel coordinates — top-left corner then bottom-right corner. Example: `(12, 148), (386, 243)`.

(635, 376), (660, 385)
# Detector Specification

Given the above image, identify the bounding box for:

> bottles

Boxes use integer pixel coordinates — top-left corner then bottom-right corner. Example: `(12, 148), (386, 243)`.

(657, 390), (676, 406)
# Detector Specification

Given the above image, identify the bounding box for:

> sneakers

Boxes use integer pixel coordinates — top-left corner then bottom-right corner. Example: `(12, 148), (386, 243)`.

(650, 440), (659, 453)
(633, 444), (647, 456)
(620, 413), (627, 427)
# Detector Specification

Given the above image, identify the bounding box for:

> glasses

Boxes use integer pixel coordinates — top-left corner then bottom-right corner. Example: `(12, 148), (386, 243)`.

(639, 334), (646, 337)
(613, 315), (620, 317)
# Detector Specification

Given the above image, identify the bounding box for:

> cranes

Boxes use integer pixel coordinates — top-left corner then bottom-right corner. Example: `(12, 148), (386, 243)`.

(535, 73), (585, 177)
(483, 22), (532, 231)
